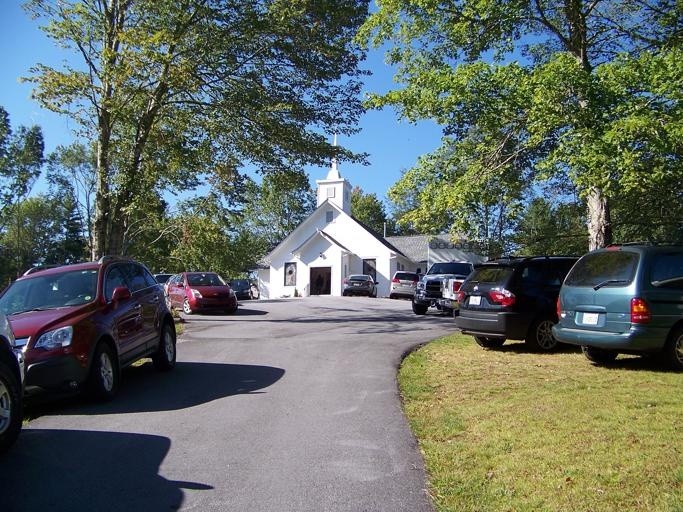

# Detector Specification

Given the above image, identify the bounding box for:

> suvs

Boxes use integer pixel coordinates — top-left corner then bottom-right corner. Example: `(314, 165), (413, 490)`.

(1, 254), (184, 395)
(409, 261), (479, 317)
(458, 251), (584, 355)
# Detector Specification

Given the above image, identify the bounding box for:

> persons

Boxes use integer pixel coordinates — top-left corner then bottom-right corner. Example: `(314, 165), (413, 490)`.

(315, 274), (324, 295)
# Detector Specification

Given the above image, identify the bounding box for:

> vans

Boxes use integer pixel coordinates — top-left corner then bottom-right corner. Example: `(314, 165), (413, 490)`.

(390, 269), (421, 300)
(551, 238), (682, 372)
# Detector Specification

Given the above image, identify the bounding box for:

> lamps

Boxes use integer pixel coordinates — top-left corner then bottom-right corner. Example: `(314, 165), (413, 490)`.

(318, 250), (322, 257)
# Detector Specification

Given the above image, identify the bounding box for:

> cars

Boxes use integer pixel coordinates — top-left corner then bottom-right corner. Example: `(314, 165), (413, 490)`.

(0, 346), (24, 456)
(154, 268), (259, 317)
(342, 273), (379, 296)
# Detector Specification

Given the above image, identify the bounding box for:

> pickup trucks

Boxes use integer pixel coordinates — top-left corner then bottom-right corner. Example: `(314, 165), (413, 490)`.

(438, 274), (465, 309)
